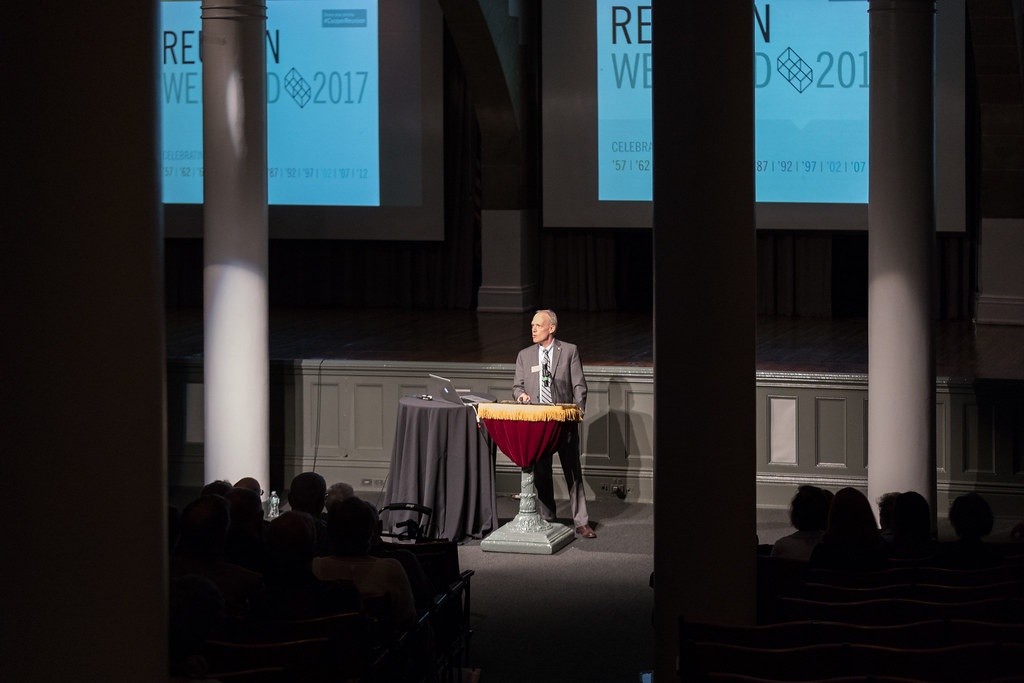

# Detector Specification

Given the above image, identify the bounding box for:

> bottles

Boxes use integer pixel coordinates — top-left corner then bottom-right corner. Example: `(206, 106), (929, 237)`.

(267, 491), (280, 518)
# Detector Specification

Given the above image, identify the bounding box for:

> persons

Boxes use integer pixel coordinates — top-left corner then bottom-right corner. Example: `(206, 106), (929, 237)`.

(512, 309), (597, 538)
(168, 472), (427, 630)
(767, 487), (995, 575)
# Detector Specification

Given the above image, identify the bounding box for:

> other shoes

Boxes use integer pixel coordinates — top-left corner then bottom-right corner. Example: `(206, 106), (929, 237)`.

(575, 524), (597, 538)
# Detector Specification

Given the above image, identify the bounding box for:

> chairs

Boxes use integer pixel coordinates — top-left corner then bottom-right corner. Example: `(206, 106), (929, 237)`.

(680, 541), (1024, 683)
(161, 539), (476, 682)
(378, 503), (433, 544)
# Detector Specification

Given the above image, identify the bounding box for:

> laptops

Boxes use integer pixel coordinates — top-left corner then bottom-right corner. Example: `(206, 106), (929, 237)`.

(429, 374), (493, 406)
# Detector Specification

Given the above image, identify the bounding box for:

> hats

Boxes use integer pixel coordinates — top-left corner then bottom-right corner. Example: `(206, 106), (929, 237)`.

(233, 478), (260, 494)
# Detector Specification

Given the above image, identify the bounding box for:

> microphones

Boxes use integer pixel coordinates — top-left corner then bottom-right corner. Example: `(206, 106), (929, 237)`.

(540, 359), (549, 387)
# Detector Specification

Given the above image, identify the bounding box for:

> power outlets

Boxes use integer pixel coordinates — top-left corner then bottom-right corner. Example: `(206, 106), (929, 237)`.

(610, 484), (624, 495)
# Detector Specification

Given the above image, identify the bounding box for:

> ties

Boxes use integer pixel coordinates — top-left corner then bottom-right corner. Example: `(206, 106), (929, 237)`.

(540, 349), (553, 405)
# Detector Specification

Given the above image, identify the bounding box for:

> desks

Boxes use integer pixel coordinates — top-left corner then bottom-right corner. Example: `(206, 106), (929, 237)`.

(379, 391), (498, 541)
